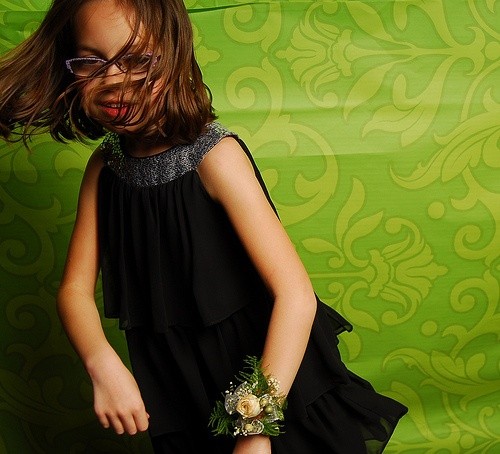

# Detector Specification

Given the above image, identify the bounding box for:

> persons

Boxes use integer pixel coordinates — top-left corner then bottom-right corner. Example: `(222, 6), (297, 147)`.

(0, 0), (409, 454)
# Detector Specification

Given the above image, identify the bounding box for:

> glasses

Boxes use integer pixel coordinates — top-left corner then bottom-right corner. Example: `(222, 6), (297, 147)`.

(64, 52), (164, 79)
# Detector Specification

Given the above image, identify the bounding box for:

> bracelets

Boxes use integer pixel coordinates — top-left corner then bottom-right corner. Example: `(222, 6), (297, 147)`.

(207, 353), (288, 441)
